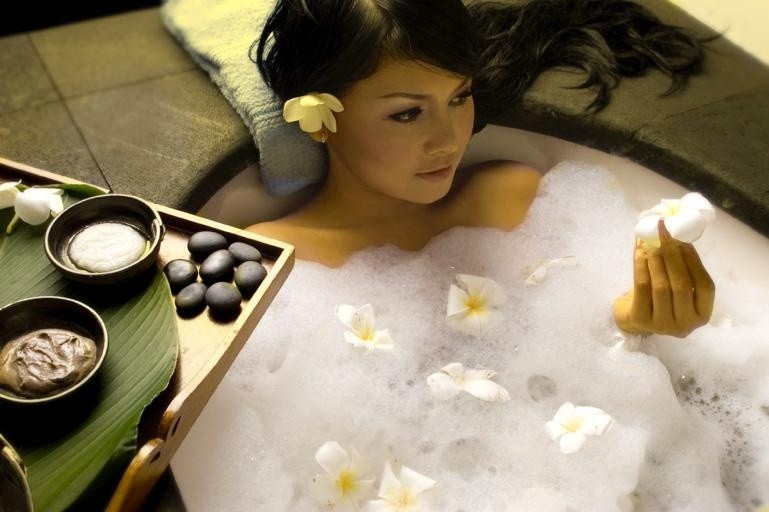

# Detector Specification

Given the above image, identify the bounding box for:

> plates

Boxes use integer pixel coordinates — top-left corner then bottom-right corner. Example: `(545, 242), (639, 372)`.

(0, 296), (110, 405)
(43, 193), (166, 288)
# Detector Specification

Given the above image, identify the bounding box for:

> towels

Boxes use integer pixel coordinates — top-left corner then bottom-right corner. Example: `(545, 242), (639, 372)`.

(158, 0), (329, 198)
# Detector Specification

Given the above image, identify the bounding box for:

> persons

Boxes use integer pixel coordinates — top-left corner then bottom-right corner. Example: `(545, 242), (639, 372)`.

(244, 1), (733, 340)
